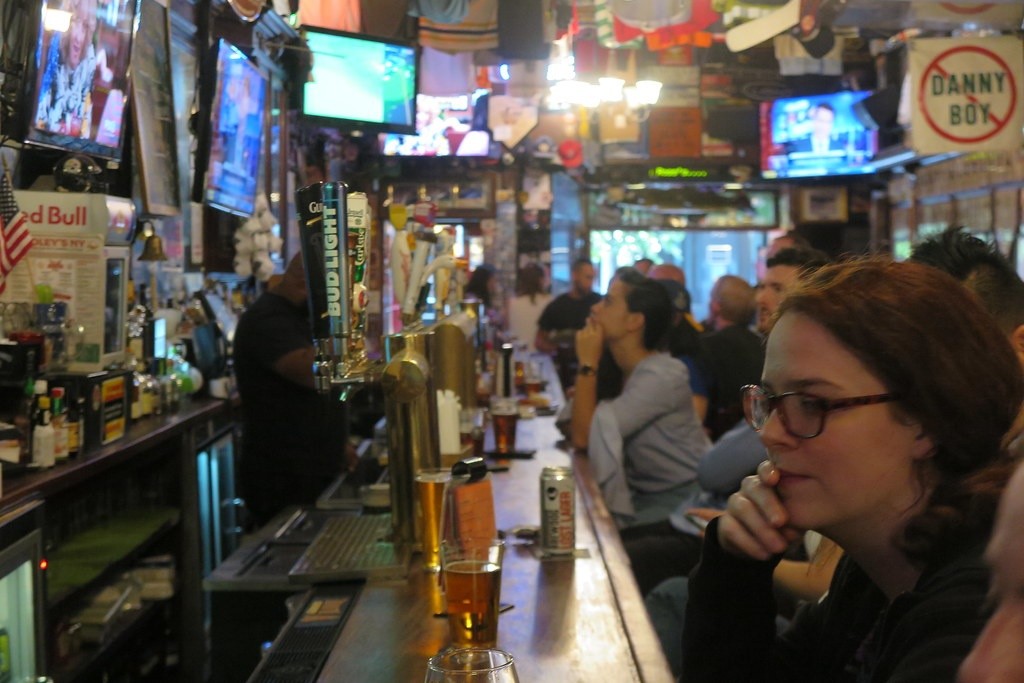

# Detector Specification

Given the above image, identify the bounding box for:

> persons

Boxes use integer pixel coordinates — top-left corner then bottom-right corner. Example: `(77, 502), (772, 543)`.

(35, 0), (98, 138)
(232, 250), (333, 514)
(786, 104), (844, 154)
(466, 220), (1024, 683)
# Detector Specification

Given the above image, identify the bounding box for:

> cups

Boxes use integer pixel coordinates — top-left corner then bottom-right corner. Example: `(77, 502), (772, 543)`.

(523, 362), (542, 394)
(441, 538), (505, 650)
(424, 646), (519, 683)
(488, 395), (519, 454)
(39, 322), (86, 368)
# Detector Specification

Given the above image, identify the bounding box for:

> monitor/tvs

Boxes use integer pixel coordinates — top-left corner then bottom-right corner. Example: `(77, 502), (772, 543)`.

(754, 83), (884, 185)
(297, 25), (421, 134)
(367, 85), (495, 160)
(191, 38), (270, 217)
(17, 0), (143, 162)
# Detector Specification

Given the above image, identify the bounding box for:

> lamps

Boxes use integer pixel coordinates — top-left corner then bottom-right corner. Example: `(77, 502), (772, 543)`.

(547, 30), (663, 124)
(132, 217), (168, 263)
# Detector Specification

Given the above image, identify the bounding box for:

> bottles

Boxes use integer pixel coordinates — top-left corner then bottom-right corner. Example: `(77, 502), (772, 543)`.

(28, 381), (85, 470)
(123, 347), (139, 402)
(154, 357), (180, 414)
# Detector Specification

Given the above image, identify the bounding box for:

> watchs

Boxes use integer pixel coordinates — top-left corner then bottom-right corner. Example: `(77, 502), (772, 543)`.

(575, 364), (597, 377)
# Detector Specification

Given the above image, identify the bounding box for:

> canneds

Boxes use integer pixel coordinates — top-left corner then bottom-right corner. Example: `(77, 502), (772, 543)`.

(539, 467), (577, 554)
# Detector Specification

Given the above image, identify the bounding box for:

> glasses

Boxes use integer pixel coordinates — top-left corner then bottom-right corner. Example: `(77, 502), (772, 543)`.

(738, 383), (896, 439)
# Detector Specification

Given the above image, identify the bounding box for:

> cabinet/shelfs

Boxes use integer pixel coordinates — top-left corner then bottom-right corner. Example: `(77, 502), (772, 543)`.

(1, 395), (241, 683)
